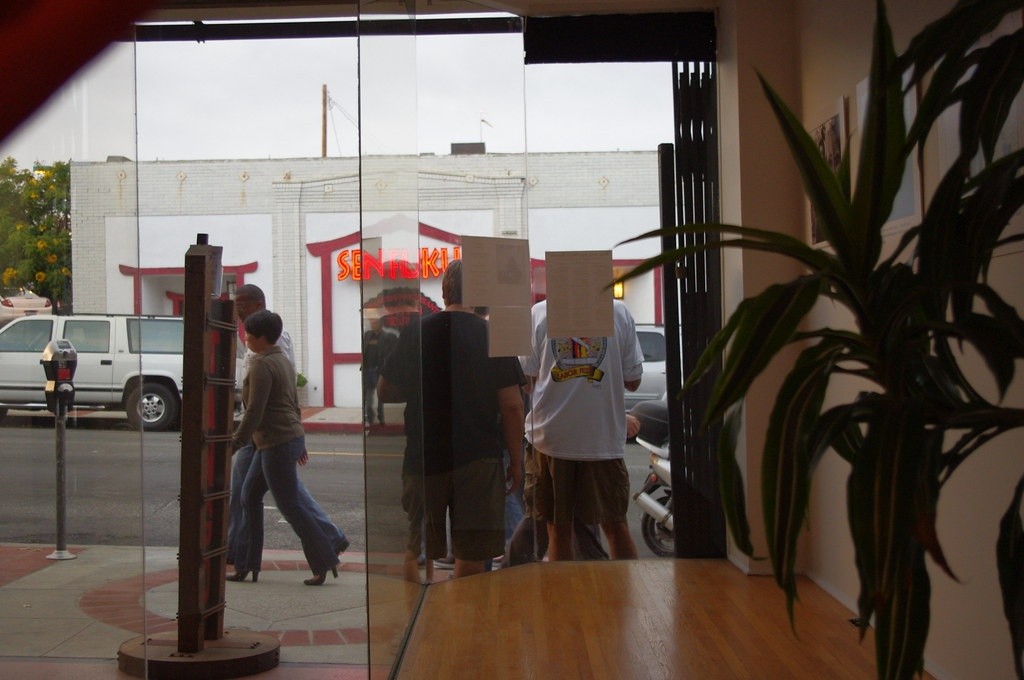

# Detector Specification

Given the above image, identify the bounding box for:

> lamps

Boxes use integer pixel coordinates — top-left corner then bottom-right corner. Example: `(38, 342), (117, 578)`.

(613, 279), (624, 298)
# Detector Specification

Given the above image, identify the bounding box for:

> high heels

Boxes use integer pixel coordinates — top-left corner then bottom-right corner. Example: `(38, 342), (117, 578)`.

(226, 569), (258, 583)
(304, 566), (339, 584)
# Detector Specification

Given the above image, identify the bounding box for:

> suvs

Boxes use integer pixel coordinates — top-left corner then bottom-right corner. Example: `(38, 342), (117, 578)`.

(626, 323), (668, 412)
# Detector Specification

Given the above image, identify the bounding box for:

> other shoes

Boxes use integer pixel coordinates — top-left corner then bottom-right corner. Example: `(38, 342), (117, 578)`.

(436, 556), (454, 568)
(336, 539), (349, 553)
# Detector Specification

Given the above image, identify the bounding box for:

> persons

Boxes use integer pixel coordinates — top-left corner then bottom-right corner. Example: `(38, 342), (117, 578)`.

(360, 315), (398, 425)
(493, 297), (647, 568)
(365, 261), (421, 618)
(231, 284), (350, 561)
(377, 260), (528, 619)
(227, 310), (341, 585)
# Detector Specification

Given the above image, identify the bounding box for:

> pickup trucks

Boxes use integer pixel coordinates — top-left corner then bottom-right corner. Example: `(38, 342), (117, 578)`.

(0, 313), (183, 433)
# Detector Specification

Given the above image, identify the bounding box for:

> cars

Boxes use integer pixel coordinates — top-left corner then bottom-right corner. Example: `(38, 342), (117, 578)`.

(0, 288), (53, 327)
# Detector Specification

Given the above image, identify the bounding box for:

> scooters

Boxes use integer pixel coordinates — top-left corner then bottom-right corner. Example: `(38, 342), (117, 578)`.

(630, 400), (675, 558)
